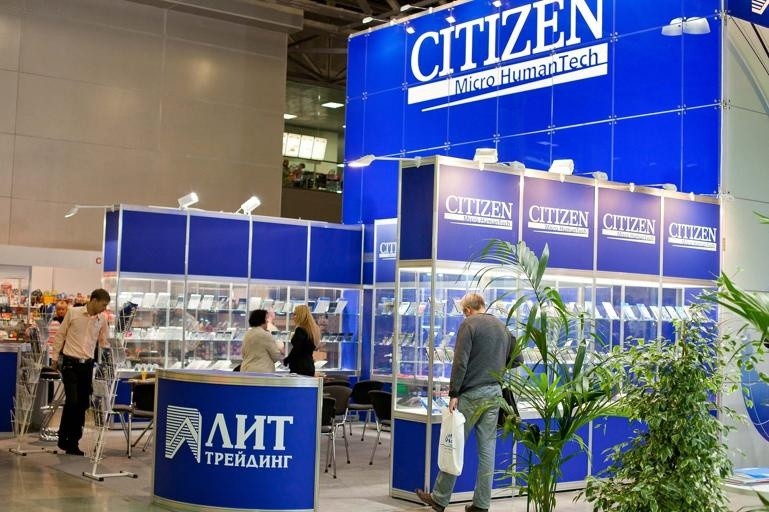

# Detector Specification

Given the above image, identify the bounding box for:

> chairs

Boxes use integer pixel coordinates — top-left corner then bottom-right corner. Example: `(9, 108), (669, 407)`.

(324, 385), (350, 467)
(128, 384), (154, 458)
(324, 381), (352, 436)
(30, 328), (66, 442)
(93, 379), (133, 455)
(321, 397), (336, 478)
(347, 381), (384, 445)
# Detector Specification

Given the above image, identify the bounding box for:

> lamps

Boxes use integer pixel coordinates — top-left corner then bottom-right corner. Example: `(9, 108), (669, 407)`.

(362, 17), (388, 25)
(65, 204), (115, 219)
(177, 191), (199, 212)
(240, 195), (261, 215)
(400, 4), (428, 11)
(473, 148), (499, 171)
(347, 154), (421, 169)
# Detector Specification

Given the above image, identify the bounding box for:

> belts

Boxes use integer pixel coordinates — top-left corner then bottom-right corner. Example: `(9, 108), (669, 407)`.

(76, 358), (85, 364)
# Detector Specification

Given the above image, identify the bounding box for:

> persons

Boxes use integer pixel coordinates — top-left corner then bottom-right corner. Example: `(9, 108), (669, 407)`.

(282, 159), (292, 188)
(169, 306), (199, 367)
(291, 161), (306, 189)
(239, 308), (282, 373)
(49, 300), (71, 324)
(284, 304), (321, 378)
(414, 291), (525, 512)
(47, 288), (112, 456)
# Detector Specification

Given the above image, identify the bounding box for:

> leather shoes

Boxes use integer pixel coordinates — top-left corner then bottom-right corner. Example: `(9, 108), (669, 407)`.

(417, 489), (445, 512)
(66, 444), (84, 455)
(465, 504), (488, 512)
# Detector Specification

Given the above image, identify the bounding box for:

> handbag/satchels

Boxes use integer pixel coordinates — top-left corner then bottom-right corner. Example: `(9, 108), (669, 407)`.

(437, 405), (466, 476)
(498, 386), (519, 428)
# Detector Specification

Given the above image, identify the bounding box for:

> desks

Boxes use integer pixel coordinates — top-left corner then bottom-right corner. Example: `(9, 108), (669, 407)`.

(716, 482), (768, 498)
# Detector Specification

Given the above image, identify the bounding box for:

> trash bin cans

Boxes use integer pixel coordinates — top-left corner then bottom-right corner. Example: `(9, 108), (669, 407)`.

(32, 366), (62, 429)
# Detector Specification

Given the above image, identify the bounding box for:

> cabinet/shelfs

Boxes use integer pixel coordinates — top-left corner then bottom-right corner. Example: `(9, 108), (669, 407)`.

(100, 204), (366, 384)
(0, 286), (80, 342)
(369, 156), (723, 508)
